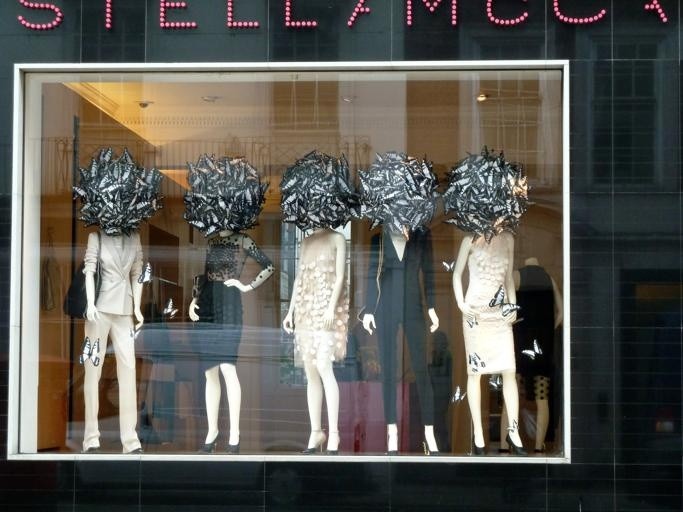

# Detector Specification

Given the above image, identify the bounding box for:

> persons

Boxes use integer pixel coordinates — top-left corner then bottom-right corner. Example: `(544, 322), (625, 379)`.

(356, 222), (444, 456)
(511, 256), (562, 455)
(188, 230), (277, 455)
(281, 226), (353, 456)
(426, 331), (454, 454)
(80, 229), (145, 455)
(452, 232), (527, 457)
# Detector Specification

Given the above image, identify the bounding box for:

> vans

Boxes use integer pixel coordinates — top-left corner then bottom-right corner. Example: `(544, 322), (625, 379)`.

(53, 322), (411, 455)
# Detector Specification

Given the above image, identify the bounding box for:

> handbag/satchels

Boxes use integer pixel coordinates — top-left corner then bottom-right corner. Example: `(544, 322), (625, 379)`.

(191, 274), (206, 298)
(357, 303), (377, 324)
(63, 231), (102, 320)
(40, 230), (61, 313)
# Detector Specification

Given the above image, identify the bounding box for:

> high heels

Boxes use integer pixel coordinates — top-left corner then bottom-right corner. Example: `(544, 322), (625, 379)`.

(387, 435), (398, 455)
(226, 435), (241, 453)
(301, 429), (326, 453)
(473, 434), (485, 455)
(506, 433), (528, 456)
(201, 432), (221, 453)
(423, 438), (440, 455)
(325, 434), (340, 455)
